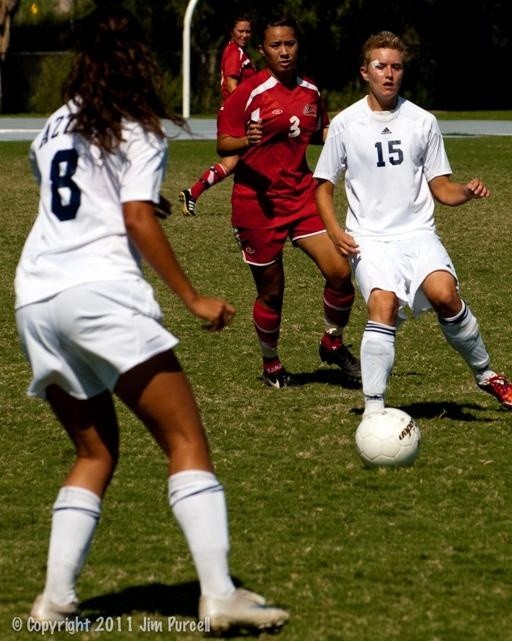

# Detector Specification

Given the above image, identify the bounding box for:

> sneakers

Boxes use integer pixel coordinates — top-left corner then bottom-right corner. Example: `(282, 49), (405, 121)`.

(179, 188), (195, 216)
(319, 344), (361, 377)
(477, 365), (512, 409)
(30, 594), (78, 623)
(198, 587), (290, 636)
(262, 356), (295, 388)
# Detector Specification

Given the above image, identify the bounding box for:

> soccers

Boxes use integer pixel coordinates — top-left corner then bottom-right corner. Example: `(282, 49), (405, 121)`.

(356, 407), (421, 468)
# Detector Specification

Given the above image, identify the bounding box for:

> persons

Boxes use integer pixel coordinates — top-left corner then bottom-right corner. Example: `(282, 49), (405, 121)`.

(179, 14), (260, 218)
(214, 14), (361, 392)
(10, 4), (289, 631)
(311, 29), (511, 417)
